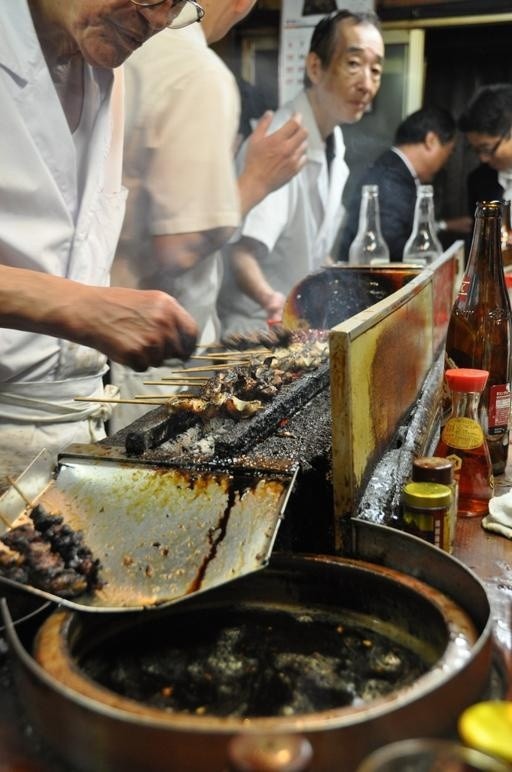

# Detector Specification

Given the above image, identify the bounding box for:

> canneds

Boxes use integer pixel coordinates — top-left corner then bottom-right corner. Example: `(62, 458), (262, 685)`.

(455, 698), (512, 771)
(401, 455), (454, 556)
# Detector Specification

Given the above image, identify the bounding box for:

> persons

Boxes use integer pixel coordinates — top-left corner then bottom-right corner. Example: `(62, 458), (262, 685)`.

(224, 12), (386, 321)
(461, 83), (510, 223)
(1, 0), (212, 507)
(103, 0), (309, 439)
(338, 103), (457, 263)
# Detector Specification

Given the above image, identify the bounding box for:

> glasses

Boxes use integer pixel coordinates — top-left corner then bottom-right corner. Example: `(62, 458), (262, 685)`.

(131, 0), (206, 30)
(467, 122), (512, 157)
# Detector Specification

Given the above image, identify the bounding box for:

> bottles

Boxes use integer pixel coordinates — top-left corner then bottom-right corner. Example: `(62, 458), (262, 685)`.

(404, 184), (446, 265)
(435, 360), (496, 519)
(347, 183), (390, 265)
(403, 457), (458, 553)
(439, 201), (512, 476)
